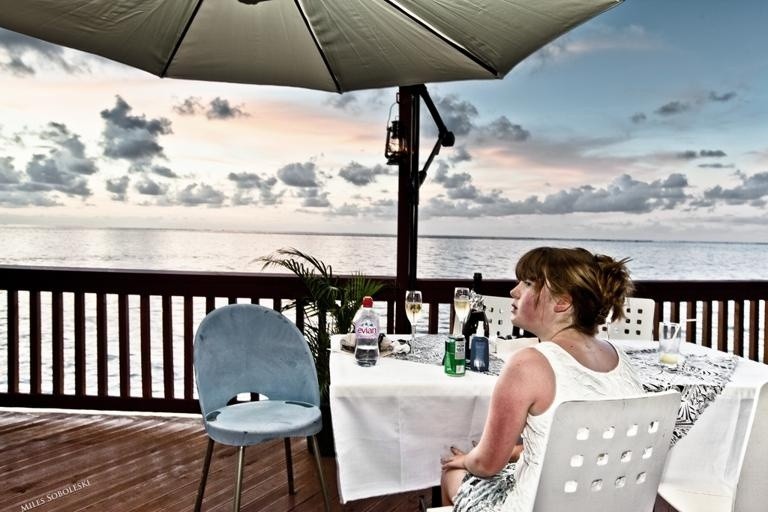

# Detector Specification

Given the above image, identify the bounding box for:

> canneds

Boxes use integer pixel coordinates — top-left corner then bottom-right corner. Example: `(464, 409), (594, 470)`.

(445, 334), (466, 376)
(470, 337), (489, 372)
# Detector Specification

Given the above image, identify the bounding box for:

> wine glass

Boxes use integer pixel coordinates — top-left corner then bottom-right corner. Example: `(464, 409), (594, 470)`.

(401, 290), (427, 348)
(454, 285), (474, 325)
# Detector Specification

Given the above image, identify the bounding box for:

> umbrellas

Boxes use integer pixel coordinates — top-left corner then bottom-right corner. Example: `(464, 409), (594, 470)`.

(0, 1), (625, 334)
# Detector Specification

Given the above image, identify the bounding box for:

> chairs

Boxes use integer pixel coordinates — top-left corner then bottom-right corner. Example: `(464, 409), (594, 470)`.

(192, 303), (334, 512)
(594, 297), (656, 341)
(450, 295), (516, 340)
(425, 389), (681, 512)
(657, 381), (768, 511)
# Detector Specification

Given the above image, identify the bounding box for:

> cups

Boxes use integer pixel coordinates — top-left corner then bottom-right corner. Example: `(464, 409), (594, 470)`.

(654, 319), (681, 367)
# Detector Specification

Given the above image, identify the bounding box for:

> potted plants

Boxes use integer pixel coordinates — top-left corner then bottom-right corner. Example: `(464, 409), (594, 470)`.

(246, 245), (387, 458)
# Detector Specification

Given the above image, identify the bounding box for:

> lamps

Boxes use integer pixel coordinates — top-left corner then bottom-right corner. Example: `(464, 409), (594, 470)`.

(384, 102), (410, 165)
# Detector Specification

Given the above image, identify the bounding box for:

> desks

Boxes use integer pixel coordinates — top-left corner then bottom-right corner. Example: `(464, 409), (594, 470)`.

(328, 333), (768, 512)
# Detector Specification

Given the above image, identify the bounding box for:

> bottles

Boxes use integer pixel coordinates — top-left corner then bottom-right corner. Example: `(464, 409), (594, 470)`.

(351, 294), (381, 367)
(467, 317), (491, 372)
(463, 271), (489, 365)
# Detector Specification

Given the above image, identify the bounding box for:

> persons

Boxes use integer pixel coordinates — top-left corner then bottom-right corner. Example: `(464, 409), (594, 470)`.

(441, 247), (649, 512)
